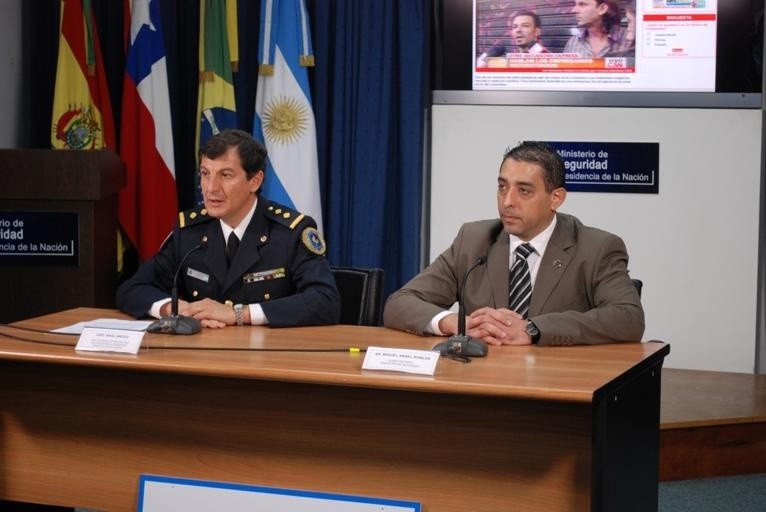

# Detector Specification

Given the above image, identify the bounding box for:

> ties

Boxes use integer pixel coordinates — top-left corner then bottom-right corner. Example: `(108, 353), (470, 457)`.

(509, 244), (533, 319)
(226, 231), (238, 266)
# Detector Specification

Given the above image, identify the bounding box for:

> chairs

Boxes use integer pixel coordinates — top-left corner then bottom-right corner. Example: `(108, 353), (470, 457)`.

(328, 262), (386, 327)
(631, 278), (644, 300)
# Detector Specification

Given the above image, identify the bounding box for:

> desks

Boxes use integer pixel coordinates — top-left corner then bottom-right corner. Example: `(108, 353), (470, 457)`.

(0, 304), (671, 510)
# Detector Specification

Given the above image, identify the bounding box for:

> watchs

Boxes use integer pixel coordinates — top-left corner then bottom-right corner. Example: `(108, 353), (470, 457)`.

(523, 317), (542, 347)
(231, 302), (247, 328)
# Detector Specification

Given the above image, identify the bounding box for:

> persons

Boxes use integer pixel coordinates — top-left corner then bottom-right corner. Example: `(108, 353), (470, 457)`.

(504, 6), (550, 53)
(382, 140), (648, 348)
(562, 1), (635, 58)
(115, 130), (343, 330)
(625, 3), (635, 43)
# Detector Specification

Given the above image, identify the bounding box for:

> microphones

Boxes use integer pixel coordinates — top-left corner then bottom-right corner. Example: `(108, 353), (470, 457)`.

(147, 241), (209, 335)
(432, 255), (489, 357)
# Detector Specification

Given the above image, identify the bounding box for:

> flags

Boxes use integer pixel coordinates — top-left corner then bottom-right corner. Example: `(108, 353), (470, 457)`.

(253, 2), (323, 221)
(52, 2), (113, 149)
(119, 0), (176, 259)
(196, 1), (239, 204)
